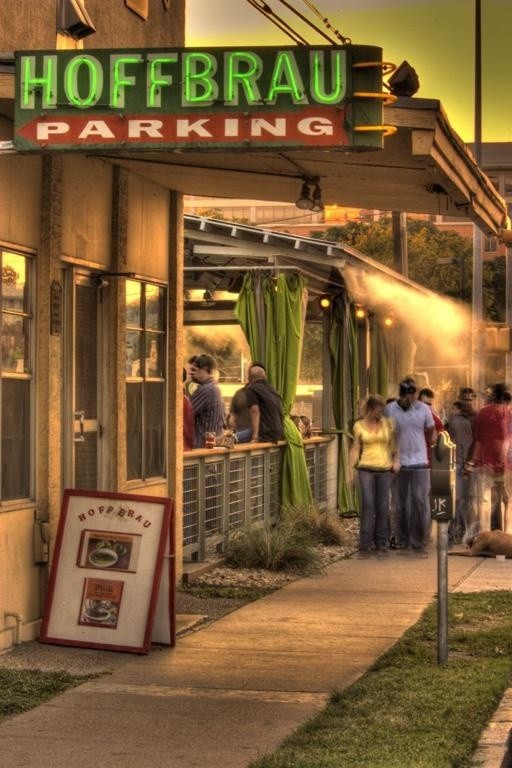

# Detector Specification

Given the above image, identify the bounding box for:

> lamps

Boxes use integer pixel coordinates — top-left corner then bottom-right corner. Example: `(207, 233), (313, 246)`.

(295, 174), (325, 214)
(382, 60), (420, 98)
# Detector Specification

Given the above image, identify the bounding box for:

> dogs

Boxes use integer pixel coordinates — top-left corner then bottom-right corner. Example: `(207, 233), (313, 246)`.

(447, 529), (512, 557)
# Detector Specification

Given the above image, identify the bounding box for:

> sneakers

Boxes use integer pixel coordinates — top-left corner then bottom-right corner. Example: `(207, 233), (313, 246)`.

(357, 537), (428, 555)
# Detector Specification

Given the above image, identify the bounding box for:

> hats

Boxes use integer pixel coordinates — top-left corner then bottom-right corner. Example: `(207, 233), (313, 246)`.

(400, 378), (416, 394)
(489, 384), (511, 401)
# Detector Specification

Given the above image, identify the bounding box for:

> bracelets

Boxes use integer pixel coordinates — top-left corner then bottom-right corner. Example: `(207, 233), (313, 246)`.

(252, 438), (258, 440)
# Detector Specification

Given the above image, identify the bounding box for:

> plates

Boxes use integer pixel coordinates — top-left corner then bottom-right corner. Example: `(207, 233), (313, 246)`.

(84, 607), (111, 621)
(89, 541), (129, 567)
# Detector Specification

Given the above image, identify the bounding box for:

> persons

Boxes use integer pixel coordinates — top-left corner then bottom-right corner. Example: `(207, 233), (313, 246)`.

(291, 415), (303, 432)
(344, 375), (512, 561)
(182, 367), (196, 452)
(187, 353), (226, 450)
(227, 363), (266, 434)
(300, 416), (312, 439)
(234, 365), (285, 445)
